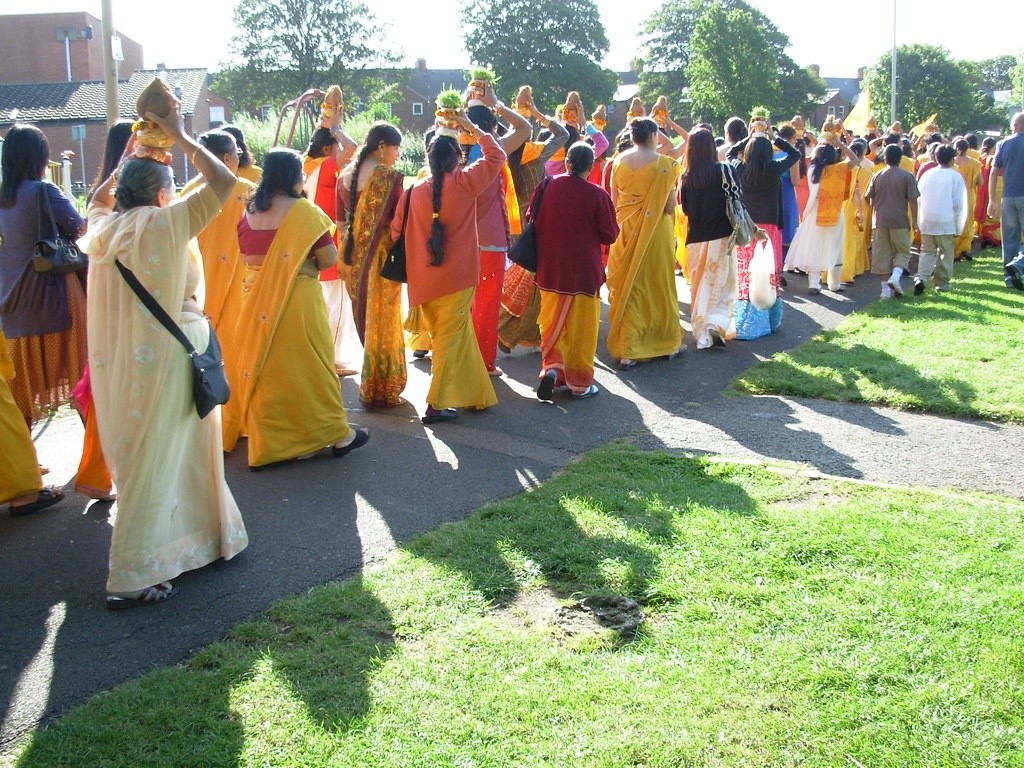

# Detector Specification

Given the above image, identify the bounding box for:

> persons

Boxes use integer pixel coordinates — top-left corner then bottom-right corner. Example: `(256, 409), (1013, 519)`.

(986, 113), (1024, 291)
(300, 78), (1024, 424)
(236, 148), (372, 471)
(0, 86), (266, 611)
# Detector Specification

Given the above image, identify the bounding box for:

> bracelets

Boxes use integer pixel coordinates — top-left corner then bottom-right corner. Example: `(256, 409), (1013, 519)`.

(469, 124), (478, 137)
(670, 123), (675, 130)
(191, 145), (204, 164)
(493, 100), (504, 114)
(754, 225), (759, 235)
(332, 130), (342, 137)
(839, 142), (843, 148)
(543, 119), (549, 126)
(113, 171), (117, 183)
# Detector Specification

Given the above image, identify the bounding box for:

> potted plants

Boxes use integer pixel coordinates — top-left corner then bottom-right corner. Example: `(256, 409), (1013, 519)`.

(748, 103), (770, 132)
(462, 64), (503, 99)
(436, 81), (465, 131)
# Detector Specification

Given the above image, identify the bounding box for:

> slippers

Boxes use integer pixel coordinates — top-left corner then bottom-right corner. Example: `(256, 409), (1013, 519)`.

(537, 374), (555, 400)
(106, 581), (181, 610)
(8, 489), (65, 516)
(422, 410), (457, 424)
(395, 397), (407, 406)
(572, 385), (599, 399)
(665, 343), (688, 360)
(332, 427), (370, 458)
(617, 357), (636, 370)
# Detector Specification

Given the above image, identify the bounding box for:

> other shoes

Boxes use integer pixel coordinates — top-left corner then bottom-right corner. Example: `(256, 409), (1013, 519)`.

(497, 338), (510, 354)
(808, 284), (821, 296)
(1005, 264), (1024, 291)
(533, 346), (541, 352)
(88, 488), (118, 501)
(781, 276), (788, 286)
(487, 366), (502, 376)
(413, 352), (424, 358)
(709, 329), (728, 349)
(881, 238), (996, 299)
(839, 284), (846, 290)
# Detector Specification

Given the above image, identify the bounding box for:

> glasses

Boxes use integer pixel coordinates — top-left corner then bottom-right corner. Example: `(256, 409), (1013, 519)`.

(296, 173), (308, 182)
(231, 148), (243, 158)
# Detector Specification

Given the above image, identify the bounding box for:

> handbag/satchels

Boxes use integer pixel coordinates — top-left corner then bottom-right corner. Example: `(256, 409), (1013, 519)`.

(720, 163), (754, 256)
(379, 184), (413, 283)
(33, 181), (86, 277)
(507, 175), (552, 272)
(190, 323), (230, 420)
(748, 233), (777, 310)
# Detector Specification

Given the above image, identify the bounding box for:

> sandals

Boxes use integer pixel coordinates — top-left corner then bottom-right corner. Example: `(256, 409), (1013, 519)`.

(335, 363), (358, 375)
